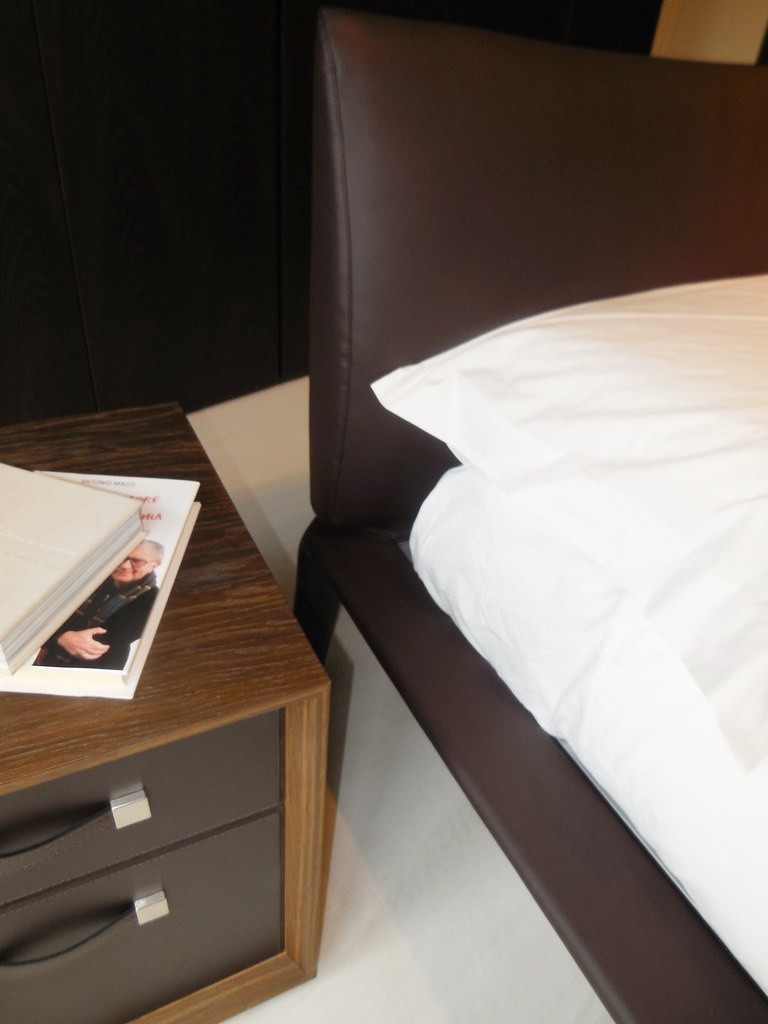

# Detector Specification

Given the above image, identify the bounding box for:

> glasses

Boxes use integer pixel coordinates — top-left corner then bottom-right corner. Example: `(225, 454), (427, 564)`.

(126, 557), (159, 567)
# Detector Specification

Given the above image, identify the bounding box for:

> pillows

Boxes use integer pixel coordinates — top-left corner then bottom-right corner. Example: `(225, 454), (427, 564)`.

(367, 273), (768, 769)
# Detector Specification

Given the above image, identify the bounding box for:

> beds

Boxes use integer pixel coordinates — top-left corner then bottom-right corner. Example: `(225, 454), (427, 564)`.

(294, 9), (768, 1023)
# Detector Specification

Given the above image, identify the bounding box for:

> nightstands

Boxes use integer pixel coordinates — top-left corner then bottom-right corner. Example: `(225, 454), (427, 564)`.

(0, 400), (330, 1023)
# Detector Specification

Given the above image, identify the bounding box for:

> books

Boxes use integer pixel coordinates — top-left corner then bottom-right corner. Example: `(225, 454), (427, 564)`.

(0, 462), (203, 700)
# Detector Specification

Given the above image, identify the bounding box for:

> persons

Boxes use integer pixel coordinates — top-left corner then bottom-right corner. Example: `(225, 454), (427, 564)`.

(33, 539), (165, 670)
